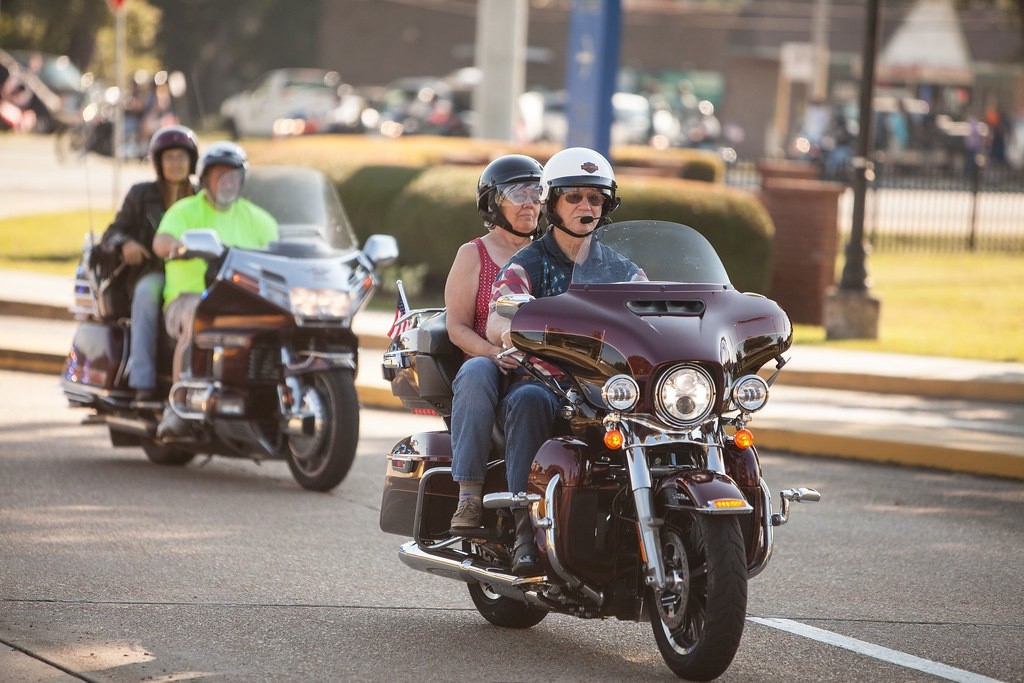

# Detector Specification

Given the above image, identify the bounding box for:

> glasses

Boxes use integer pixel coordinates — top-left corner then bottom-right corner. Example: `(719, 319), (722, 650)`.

(500, 189), (541, 204)
(559, 191), (605, 206)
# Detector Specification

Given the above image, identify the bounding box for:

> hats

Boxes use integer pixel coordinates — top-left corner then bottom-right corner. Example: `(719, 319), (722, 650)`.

(198, 141), (248, 182)
(149, 125), (198, 179)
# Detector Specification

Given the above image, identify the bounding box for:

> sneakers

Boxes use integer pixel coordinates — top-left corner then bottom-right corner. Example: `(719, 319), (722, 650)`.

(156, 402), (188, 437)
(451, 497), (488, 528)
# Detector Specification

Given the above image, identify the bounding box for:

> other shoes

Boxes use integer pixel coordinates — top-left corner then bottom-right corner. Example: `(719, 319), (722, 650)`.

(512, 509), (538, 575)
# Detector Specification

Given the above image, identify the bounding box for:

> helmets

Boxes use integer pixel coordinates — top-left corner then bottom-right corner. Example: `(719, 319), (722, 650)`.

(476, 154), (544, 211)
(539, 147), (618, 210)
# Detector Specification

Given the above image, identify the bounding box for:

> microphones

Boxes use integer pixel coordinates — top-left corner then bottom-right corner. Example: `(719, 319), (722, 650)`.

(580, 216), (608, 223)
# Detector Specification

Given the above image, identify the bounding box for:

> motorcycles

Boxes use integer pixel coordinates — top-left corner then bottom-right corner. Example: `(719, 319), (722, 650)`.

(380, 220), (821, 682)
(60, 163), (409, 497)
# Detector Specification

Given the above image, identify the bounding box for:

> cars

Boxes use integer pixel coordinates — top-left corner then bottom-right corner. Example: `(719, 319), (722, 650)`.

(0, 44), (1024, 211)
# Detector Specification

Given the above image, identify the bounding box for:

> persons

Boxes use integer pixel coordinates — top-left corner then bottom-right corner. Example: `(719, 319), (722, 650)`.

(439, 154), (548, 534)
(485, 147), (651, 582)
(152, 141), (281, 439)
(101, 126), (202, 406)
